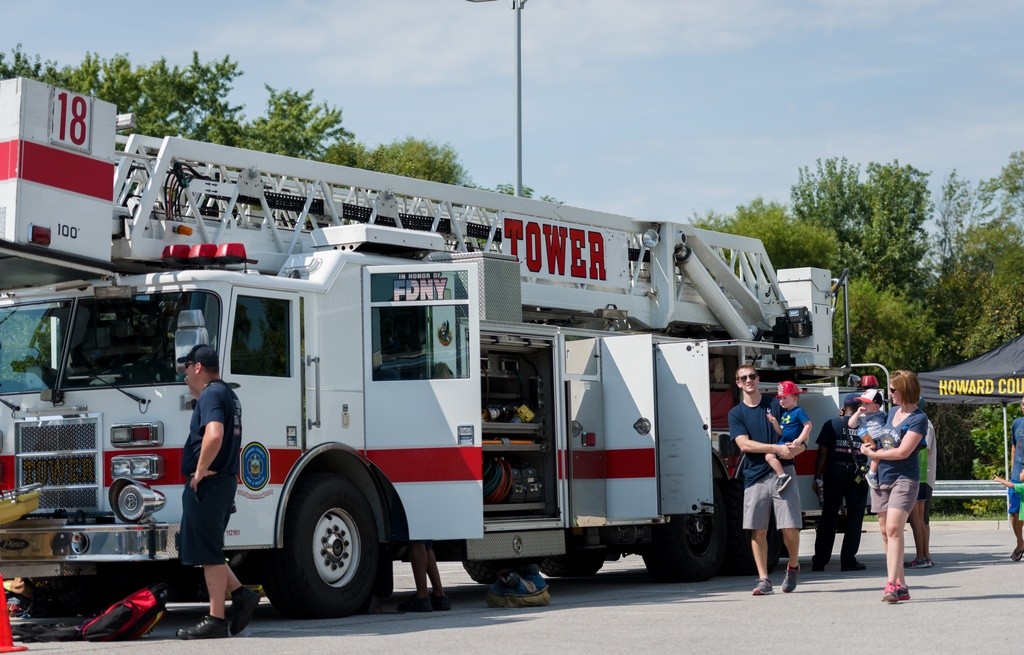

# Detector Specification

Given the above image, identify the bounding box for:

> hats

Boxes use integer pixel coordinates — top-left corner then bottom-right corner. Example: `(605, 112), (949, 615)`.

(775, 381), (805, 397)
(845, 394), (860, 405)
(855, 388), (882, 404)
(177, 344), (218, 367)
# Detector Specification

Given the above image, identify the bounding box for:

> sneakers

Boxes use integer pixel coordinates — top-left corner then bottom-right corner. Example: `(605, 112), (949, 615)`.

(904, 558), (932, 568)
(896, 584), (910, 600)
(230, 590), (260, 635)
(881, 583), (899, 602)
(753, 579), (774, 595)
(781, 560), (800, 593)
(176, 616), (228, 639)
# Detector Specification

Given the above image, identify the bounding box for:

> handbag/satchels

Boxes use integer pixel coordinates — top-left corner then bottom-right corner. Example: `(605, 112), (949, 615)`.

(485, 571), (549, 608)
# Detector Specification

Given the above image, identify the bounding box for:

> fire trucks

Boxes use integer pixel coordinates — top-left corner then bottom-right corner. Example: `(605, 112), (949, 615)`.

(0, 77), (893, 621)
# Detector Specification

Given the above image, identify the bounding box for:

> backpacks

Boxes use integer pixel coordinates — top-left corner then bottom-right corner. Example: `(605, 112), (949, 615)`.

(82, 584), (168, 640)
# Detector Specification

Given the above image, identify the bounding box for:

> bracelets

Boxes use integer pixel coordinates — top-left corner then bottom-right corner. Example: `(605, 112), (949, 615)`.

(801, 442), (807, 448)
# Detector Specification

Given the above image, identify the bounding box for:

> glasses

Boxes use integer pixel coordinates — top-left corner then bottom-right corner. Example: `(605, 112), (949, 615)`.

(737, 372), (757, 383)
(184, 361), (200, 368)
(890, 388), (897, 394)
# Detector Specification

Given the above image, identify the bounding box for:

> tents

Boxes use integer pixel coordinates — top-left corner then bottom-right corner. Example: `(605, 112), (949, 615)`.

(920, 337), (1024, 494)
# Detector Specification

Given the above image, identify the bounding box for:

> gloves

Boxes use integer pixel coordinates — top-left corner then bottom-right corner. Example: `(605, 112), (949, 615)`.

(812, 479), (823, 495)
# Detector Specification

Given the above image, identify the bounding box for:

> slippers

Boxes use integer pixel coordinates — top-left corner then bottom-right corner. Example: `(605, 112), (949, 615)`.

(1011, 545), (1024, 561)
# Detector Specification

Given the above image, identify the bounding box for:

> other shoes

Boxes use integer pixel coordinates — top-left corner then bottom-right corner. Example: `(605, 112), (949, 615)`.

(865, 473), (879, 490)
(431, 595), (452, 612)
(776, 475), (792, 492)
(812, 560), (826, 571)
(397, 598), (433, 612)
(841, 560), (866, 571)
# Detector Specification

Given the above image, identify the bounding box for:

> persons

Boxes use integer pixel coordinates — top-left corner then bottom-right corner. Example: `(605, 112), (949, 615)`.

(728, 364), (809, 595)
(848, 389), (902, 489)
(994, 468), (1024, 522)
(178, 343), (260, 639)
(765, 380), (813, 491)
(811, 393), (869, 572)
(373, 334), (450, 613)
(860, 370), (928, 603)
(3, 575), (86, 616)
(1007, 398), (1024, 561)
(903, 396), (936, 569)
